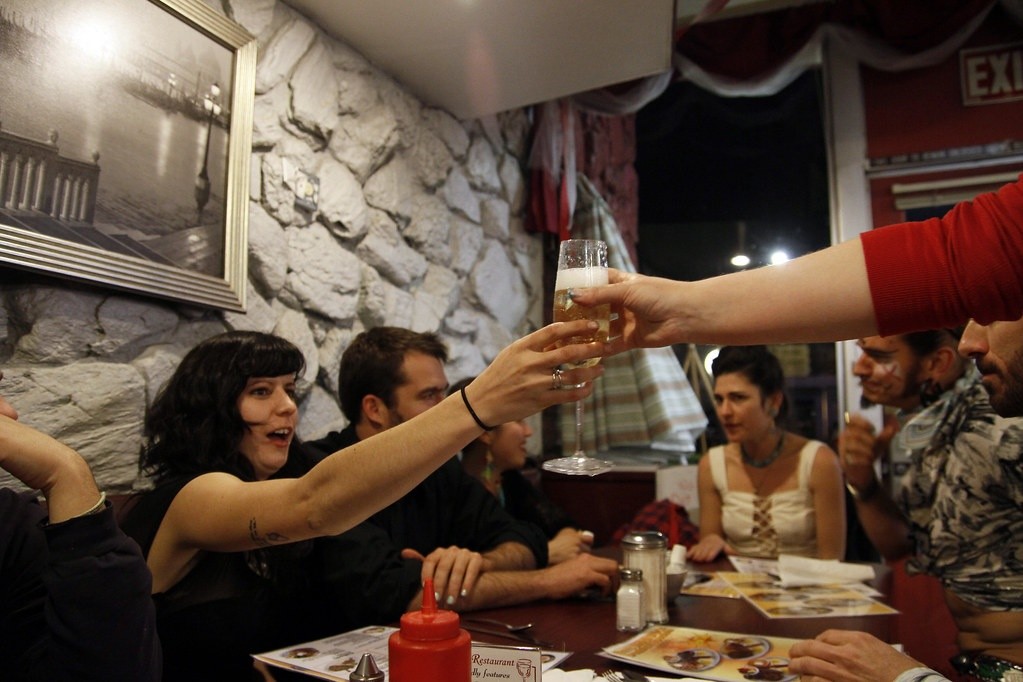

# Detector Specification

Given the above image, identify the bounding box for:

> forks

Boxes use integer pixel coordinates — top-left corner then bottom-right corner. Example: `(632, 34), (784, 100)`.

(602, 669), (623, 682)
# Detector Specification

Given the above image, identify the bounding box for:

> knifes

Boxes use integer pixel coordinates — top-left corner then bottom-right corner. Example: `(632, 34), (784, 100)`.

(461, 624), (557, 648)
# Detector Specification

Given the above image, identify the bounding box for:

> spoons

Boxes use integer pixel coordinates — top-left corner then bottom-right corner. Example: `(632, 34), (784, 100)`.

(622, 670), (648, 682)
(466, 617), (534, 630)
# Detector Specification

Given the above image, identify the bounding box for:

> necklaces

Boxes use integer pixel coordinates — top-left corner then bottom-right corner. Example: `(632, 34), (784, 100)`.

(740, 434), (784, 467)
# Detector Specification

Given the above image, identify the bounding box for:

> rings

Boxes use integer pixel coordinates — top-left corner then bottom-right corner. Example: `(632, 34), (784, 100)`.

(552, 370), (562, 389)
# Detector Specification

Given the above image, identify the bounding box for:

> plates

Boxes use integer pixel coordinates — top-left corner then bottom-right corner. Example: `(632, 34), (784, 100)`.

(745, 656), (797, 682)
(718, 637), (770, 661)
(670, 647), (720, 671)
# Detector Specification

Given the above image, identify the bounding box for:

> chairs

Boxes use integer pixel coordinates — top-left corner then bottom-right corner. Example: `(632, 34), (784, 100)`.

(543, 471), (657, 548)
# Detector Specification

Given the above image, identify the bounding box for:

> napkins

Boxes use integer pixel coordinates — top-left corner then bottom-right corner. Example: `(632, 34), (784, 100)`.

(542, 667), (714, 682)
(774, 553), (876, 587)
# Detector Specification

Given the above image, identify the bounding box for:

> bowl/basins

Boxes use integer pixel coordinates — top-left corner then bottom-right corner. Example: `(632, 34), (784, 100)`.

(668, 569), (686, 600)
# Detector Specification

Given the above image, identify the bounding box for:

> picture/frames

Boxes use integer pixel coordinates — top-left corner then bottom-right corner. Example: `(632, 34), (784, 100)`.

(0, 0), (258, 315)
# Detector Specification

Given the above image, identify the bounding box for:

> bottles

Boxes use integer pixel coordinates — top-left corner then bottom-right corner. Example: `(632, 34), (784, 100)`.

(616, 568), (646, 633)
(350, 653), (385, 682)
(389, 577), (472, 682)
(621, 531), (670, 625)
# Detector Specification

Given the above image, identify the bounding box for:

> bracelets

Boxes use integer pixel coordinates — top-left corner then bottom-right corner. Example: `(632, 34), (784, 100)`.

(461, 384), (493, 431)
(845, 478), (879, 504)
(77, 492), (106, 516)
(894, 667), (944, 682)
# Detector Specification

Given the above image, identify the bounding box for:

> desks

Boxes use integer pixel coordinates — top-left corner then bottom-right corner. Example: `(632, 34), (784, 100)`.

(251, 542), (894, 682)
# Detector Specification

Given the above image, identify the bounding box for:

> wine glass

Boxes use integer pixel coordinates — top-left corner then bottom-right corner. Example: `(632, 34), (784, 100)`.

(541, 239), (615, 477)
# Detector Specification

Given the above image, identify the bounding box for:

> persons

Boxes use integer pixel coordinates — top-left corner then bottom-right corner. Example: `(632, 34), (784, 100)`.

(0, 396), (163, 682)
(567, 172), (1023, 356)
(685, 347), (847, 563)
(839, 328), (1023, 667)
(307, 327), (621, 628)
(786, 319), (1023, 682)
(448, 376), (596, 569)
(120, 320), (604, 682)
